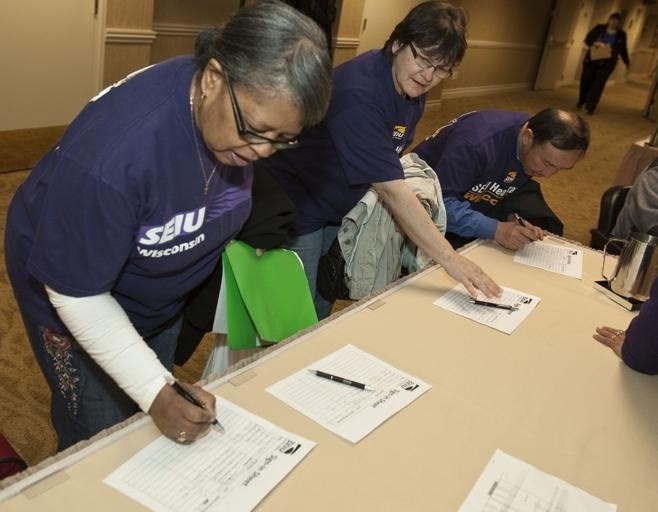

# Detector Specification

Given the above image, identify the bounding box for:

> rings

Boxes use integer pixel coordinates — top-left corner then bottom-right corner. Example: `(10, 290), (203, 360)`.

(177, 428), (190, 443)
(615, 332), (619, 336)
(619, 330), (623, 333)
(611, 332), (615, 340)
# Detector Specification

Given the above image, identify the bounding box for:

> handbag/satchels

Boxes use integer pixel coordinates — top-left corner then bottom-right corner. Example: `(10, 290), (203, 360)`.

(318, 236), (408, 301)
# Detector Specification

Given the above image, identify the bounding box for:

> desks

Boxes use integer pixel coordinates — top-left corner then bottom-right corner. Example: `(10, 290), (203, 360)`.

(2, 229), (657, 512)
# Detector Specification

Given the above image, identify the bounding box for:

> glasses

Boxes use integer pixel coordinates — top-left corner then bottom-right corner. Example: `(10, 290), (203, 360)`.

(223, 70), (303, 149)
(407, 40), (453, 80)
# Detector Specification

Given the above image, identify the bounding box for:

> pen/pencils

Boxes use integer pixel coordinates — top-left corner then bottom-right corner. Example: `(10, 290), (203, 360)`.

(317, 371), (374, 390)
(470, 300), (513, 312)
(166, 377), (227, 432)
(514, 213), (536, 247)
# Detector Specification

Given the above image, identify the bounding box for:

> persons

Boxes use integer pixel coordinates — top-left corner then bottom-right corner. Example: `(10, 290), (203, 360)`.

(592, 266), (657, 375)
(575, 10), (631, 114)
(412, 109), (588, 254)
(605, 158), (658, 256)
(251, 1), (503, 323)
(6, 1), (336, 455)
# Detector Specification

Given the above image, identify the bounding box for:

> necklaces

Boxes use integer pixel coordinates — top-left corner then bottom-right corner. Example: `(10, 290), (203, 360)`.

(190, 76), (220, 197)
